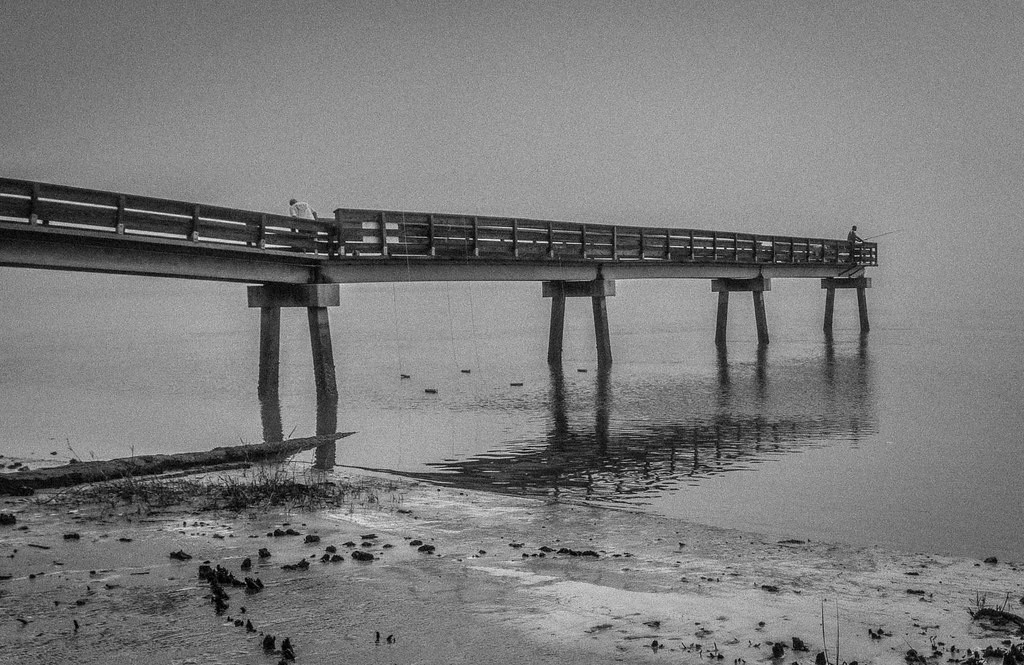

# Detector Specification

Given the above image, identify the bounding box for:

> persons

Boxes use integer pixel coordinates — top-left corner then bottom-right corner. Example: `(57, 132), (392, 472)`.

(289, 197), (321, 254)
(845, 224), (865, 265)
